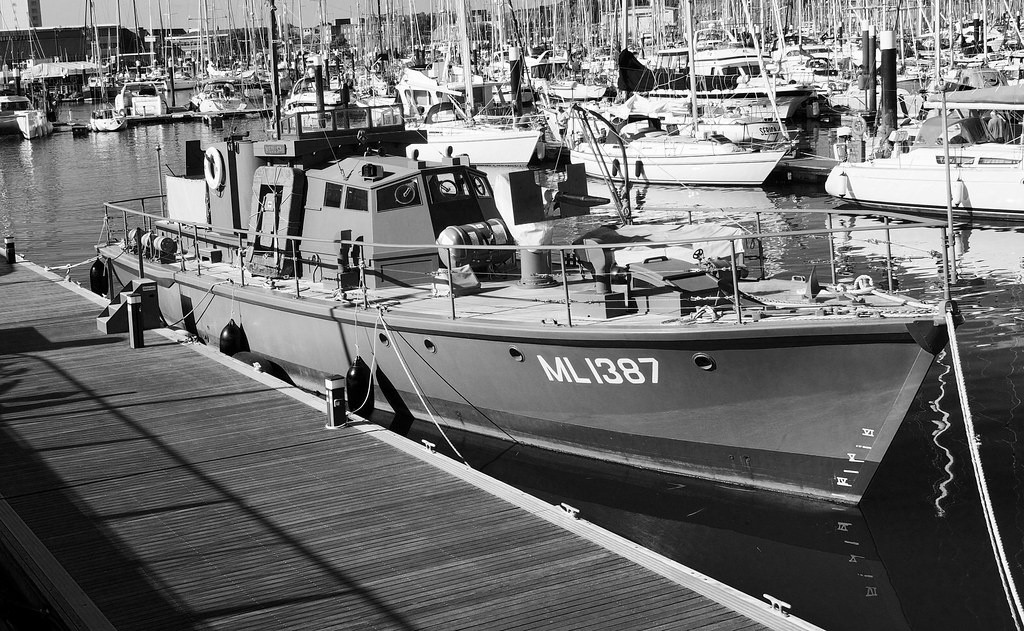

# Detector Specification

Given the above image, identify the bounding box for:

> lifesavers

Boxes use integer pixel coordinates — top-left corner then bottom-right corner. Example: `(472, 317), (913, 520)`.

(850, 116), (869, 136)
(202, 146), (223, 192)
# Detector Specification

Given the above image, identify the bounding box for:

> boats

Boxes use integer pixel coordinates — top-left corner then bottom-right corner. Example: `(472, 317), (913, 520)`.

(0, 0), (1024, 214)
(89, 64), (961, 509)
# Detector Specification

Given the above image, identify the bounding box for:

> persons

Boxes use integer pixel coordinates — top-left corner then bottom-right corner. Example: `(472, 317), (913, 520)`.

(393, 48), (400, 62)
(858, 65), (867, 80)
(531, 42), (545, 59)
(987, 111), (1006, 143)
(903, 39), (913, 57)
